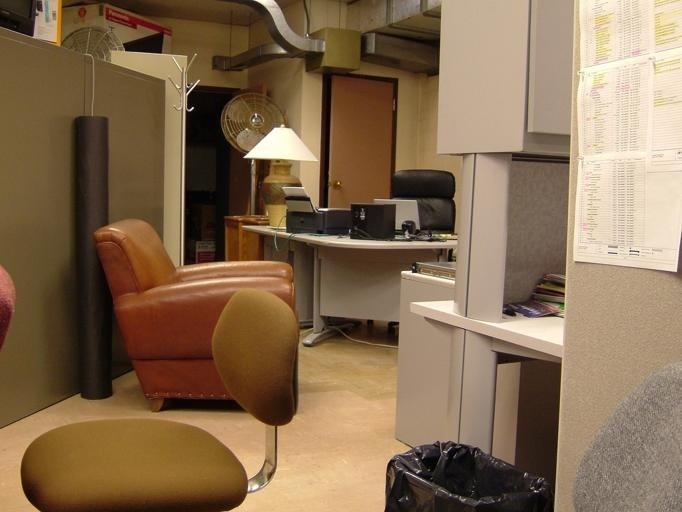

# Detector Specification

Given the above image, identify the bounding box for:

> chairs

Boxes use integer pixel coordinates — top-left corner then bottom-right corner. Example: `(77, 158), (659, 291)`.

(391, 170), (457, 234)
(20, 286), (299, 512)
(92, 219), (297, 413)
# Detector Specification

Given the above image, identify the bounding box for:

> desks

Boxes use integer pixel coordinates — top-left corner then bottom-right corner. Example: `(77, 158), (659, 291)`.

(242, 224), (458, 347)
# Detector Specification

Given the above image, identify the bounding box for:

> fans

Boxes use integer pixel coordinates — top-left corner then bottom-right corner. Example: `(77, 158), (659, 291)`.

(220, 92), (288, 216)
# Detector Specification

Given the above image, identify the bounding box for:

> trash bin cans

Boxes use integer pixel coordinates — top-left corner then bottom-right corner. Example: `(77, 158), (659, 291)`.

(392, 441), (553, 512)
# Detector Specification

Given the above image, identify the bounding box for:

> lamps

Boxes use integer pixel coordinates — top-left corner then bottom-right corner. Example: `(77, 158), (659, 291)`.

(241, 123), (319, 230)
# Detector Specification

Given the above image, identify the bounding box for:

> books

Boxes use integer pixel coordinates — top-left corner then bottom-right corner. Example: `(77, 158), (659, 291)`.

(502, 271), (566, 324)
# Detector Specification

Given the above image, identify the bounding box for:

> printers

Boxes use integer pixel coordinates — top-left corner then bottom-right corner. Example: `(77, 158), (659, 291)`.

(284, 196), (352, 235)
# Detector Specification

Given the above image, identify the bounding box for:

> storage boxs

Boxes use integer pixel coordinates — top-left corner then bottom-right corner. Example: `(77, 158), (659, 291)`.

(62, 3), (173, 53)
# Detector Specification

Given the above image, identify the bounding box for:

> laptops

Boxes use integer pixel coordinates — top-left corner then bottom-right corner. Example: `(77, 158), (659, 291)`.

(373, 198), (421, 234)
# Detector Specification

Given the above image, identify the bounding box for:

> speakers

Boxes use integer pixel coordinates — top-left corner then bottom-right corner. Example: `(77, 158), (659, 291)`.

(402, 220), (416, 234)
(350, 203), (396, 240)
